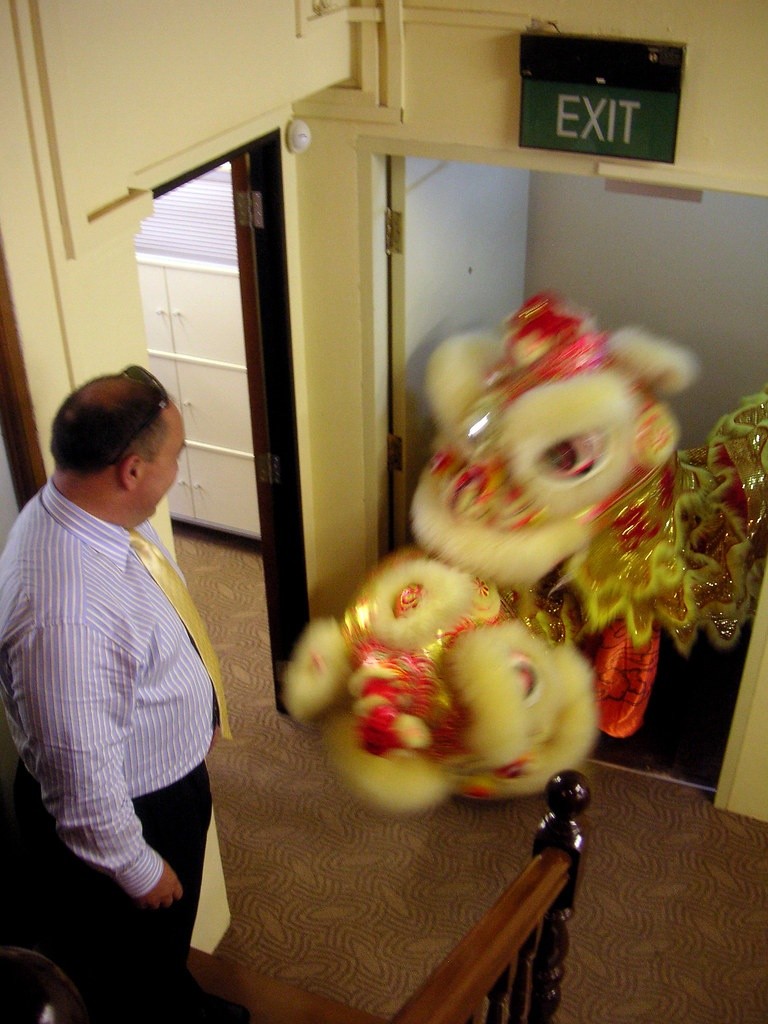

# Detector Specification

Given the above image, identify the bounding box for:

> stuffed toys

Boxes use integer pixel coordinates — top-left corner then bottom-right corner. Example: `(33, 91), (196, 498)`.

(280, 294), (768, 815)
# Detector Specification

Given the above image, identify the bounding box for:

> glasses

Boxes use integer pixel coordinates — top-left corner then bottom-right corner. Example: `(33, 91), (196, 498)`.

(108, 366), (167, 464)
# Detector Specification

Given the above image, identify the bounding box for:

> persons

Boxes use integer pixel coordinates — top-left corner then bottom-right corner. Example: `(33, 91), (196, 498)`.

(0, 365), (253, 1024)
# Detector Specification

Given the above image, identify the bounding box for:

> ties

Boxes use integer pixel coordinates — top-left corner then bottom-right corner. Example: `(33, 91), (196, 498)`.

(127, 528), (232, 740)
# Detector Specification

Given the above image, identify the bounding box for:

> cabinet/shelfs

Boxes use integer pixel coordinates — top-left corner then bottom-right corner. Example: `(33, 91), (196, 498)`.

(135, 255), (260, 543)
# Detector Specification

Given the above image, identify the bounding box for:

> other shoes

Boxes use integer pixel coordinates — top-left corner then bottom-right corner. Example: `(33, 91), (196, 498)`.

(186, 992), (250, 1024)
